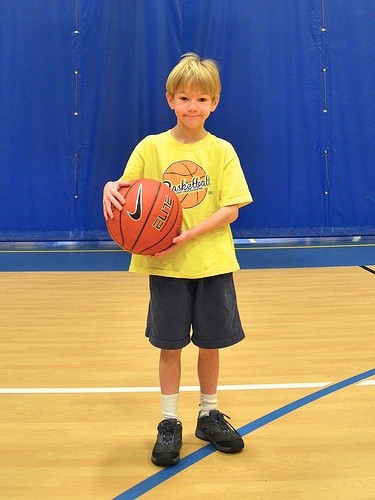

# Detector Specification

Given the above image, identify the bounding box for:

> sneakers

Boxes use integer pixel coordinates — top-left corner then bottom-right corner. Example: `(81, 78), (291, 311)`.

(151, 418), (183, 467)
(195, 409), (244, 454)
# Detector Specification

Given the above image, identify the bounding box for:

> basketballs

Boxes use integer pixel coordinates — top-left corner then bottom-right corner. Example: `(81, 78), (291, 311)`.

(105, 178), (183, 256)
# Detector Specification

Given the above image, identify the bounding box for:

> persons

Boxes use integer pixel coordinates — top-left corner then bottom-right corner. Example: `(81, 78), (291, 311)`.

(102, 51), (254, 468)
